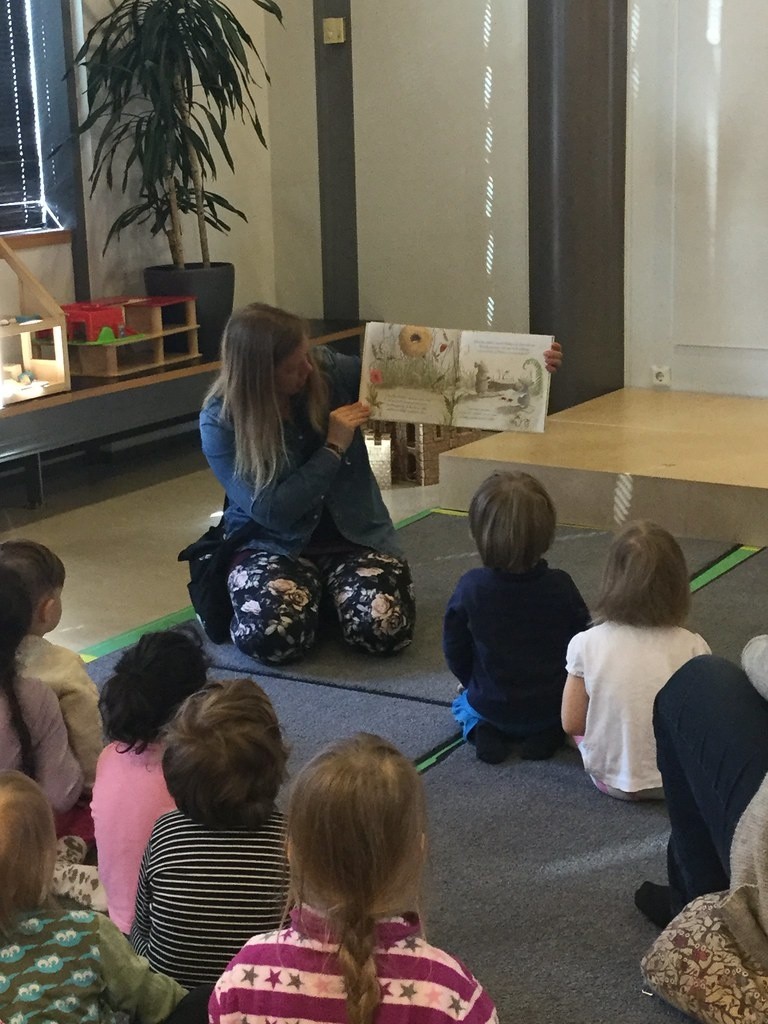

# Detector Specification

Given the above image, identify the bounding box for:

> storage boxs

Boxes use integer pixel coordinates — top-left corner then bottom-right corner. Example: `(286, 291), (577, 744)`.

(33, 296), (203, 377)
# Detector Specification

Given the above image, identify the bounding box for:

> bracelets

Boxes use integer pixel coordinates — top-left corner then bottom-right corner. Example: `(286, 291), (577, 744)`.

(323, 442), (346, 459)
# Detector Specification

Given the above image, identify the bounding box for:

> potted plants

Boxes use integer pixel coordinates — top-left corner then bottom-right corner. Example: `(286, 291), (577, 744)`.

(45, 0), (286, 357)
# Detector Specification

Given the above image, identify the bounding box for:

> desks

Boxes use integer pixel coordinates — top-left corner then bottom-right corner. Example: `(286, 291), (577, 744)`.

(0, 317), (388, 510)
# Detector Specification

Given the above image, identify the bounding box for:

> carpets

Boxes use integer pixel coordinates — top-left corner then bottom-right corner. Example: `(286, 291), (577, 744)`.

(82, 639), (463, 814)
(168, 513), (745, 715)
(405, 742), (696, 1024)
(681, 544), (768, 670)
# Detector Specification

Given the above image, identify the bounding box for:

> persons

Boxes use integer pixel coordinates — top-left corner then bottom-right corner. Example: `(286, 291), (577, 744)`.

(199, 302), (563, 667)
(441, 469), (594, 765)
(0, 540), (498, 1024)
(561, 520), (768, 1024)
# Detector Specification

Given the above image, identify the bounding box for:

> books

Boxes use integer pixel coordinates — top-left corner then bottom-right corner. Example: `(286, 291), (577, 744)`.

(357, 321), (555, 433)
